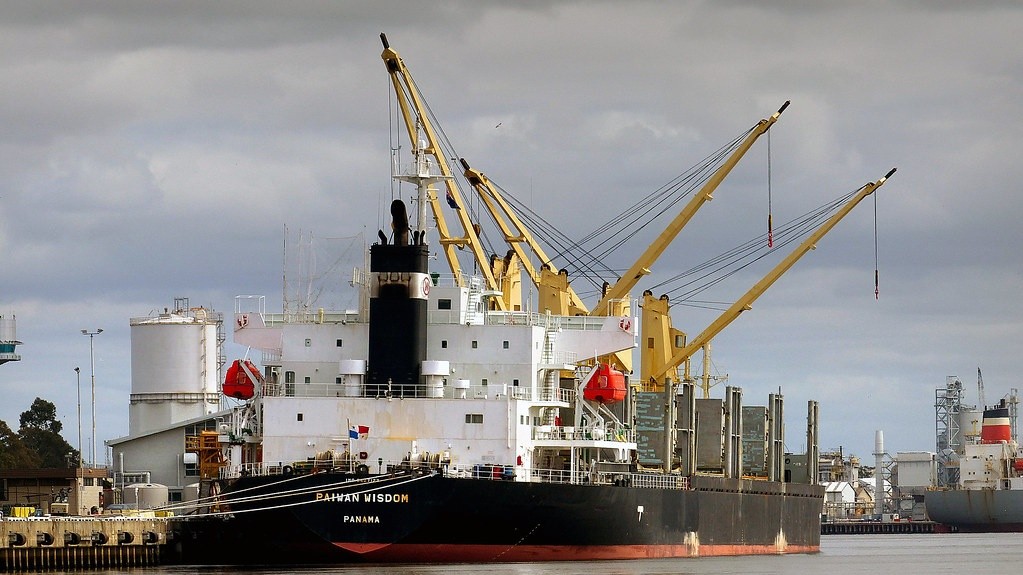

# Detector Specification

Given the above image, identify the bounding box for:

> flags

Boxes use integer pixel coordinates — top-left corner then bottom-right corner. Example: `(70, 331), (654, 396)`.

(349, 422), (369, 440)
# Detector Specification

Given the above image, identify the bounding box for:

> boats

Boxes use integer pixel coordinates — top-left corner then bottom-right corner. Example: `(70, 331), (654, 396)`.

(925, 368), (1023, 526)
(151, 29), (908, 559)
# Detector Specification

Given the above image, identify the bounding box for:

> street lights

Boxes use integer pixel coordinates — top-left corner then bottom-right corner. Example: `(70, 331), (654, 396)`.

(86, 437), (92, 466)
(74, 366), (84, 470)
(81, 328), (104, 467)
